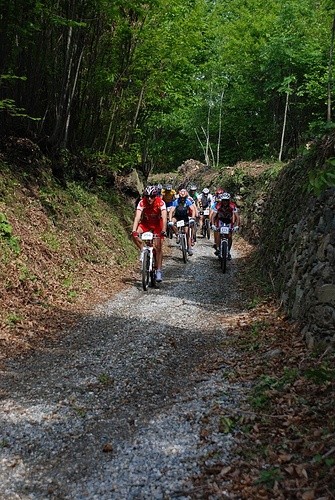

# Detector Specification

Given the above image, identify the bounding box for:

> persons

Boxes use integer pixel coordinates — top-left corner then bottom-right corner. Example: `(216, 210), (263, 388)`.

(157, 183), (241, 261)
(131, 186), (167, 282)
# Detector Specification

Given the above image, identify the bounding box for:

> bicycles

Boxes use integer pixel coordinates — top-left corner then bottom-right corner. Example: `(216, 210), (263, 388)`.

(210, 226), (239, 273)
(129, 231), (167, 291)
(166, 208), (210, 263)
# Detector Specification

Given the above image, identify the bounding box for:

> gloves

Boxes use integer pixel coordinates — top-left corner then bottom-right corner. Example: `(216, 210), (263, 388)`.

(233, 225), (239, 232)
(168, 222), (173, 227)
(189, 219), (194, 224)
(211, 225), (217, 232)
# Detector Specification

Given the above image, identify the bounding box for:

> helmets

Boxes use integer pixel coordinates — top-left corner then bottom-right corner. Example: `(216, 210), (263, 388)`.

(178, 190), (188, 198)
(190, 185), (197, 191)
(144, 186), (158, 196)
(202, 188), (210, 194)
(216, 188), (223, 194)
(157, 183), (163, 190)
(166, 186), (172, 191)
(221, 193), (231, 200)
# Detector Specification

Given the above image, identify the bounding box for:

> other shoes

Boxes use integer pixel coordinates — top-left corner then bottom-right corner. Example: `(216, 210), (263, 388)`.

(227, 254), (232, 260)
(191, 239), (195, 246)
(187, 248), (193, 255)
(214, 249), (220, 255)
(139, 251), (144, 262)
(176, 234), (181, 244)
(156, 272), (162, 282)
